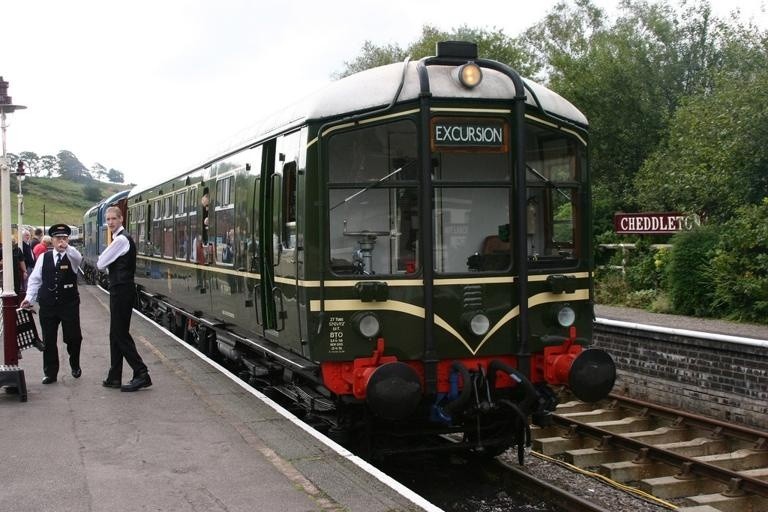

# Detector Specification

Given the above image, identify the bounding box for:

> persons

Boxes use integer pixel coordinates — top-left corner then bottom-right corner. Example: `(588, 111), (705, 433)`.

(96, 205), (152, 392)
(132, 225), (267, 300)
(201, 193), (208, 226)
(0, 222), (85, 385)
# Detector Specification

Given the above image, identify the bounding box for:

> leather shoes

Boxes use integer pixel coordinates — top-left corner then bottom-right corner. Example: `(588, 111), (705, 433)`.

(41, 376), (57, 384)
(121, 372), (153, 392)
(71, 365), (82, 378)
(102, 377), (122, 388)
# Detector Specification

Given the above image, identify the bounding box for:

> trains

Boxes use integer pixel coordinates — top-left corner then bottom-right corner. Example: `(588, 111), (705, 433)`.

(22, 224), (84, 243)
(80, 39), (619, 495)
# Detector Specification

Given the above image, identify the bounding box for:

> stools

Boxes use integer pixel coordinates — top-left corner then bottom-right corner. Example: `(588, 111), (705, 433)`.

(0, 307), (48, 402)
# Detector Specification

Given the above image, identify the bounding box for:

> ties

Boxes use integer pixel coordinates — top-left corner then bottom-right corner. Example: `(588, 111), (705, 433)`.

(56, 253), (62, 268)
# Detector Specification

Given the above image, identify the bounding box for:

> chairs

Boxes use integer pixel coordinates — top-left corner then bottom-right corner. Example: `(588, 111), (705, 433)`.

(482, 236), (512, 270)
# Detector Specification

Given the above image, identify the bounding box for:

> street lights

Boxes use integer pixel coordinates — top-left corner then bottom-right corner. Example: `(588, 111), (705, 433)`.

(11, 160), (29, 254)
(0, 77), (28, 366)
(40, 201), (48, 233)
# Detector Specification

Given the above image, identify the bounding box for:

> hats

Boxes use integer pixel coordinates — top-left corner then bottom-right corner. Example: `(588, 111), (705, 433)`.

(48, 223), (71, 238)
